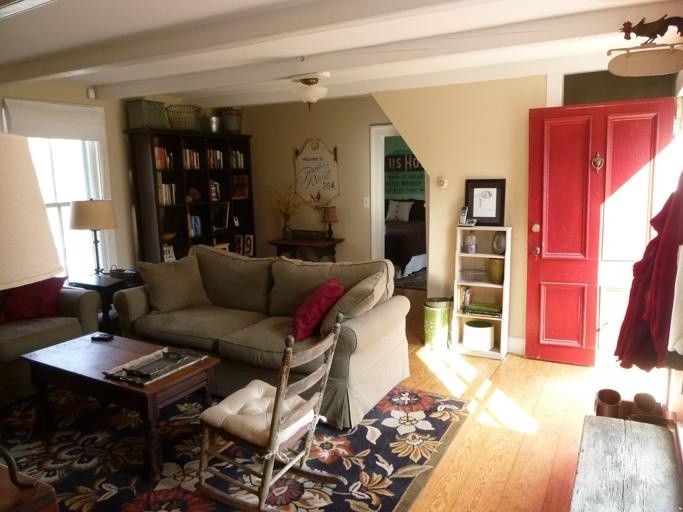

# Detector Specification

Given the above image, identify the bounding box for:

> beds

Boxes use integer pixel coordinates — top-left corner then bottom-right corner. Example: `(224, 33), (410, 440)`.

(383, 195), (428, 280)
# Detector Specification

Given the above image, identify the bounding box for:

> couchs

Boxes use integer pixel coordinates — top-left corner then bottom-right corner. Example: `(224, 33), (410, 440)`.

(1, 278), (98, 415)
(109, 242), (414, 432)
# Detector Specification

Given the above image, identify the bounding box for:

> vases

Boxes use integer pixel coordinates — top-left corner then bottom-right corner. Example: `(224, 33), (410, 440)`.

(279, 214), (292, 240)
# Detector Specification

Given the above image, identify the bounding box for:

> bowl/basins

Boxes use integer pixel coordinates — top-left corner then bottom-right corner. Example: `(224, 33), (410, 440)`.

(459, 269), (488, 282)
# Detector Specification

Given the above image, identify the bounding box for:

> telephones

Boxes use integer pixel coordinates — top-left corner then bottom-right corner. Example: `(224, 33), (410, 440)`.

(457, 206), (477, 227)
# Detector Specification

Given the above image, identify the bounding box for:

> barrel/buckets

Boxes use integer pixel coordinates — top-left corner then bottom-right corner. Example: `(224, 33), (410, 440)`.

(634, 393), (657, 413)
(421, 296), (450, 350)
(200, 116), (221, 133)
(493, 232), (505, 254)
(222, 110), (241, 133)
(595, 389), (621, 417)
(465, 230), (477, 253)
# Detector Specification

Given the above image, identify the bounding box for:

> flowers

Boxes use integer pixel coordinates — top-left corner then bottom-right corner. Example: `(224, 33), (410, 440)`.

(273, 184), (306, 217)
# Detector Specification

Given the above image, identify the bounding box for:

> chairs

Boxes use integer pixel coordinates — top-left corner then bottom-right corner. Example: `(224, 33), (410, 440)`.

(194, 312), (344, 511)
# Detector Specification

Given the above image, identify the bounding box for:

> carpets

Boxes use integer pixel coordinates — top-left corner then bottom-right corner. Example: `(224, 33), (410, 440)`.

(1, 339), (468, 512)
(394, 267), (429, 293)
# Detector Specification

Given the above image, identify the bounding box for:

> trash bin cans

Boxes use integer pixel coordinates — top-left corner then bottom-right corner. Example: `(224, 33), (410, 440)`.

(423, 297), (453, 348)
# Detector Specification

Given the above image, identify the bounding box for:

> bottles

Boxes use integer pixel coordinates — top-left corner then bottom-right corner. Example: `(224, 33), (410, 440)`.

(490, 231), (505, 255)
(464, 229), (478, 254)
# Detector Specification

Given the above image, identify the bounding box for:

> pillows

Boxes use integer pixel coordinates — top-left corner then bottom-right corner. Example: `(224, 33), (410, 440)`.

(290, 274), (341, 344)
(3, 268), (63, 322)
(320, 268), (387, 344)
(385, 198), (414, 225)
(137, 255), (212, 316)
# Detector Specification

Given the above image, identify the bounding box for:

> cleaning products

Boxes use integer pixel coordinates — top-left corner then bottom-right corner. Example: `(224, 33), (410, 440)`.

(459, 285), (472, 306)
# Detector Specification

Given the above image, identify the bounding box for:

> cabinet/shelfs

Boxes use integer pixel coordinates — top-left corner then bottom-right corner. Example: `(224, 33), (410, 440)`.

(120, 124), (258, 263)
(450, 223), (516, 361)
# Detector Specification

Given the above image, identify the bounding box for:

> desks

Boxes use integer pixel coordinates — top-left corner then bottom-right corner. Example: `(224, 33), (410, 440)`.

(15, 331), (223, 488)
(571, 412), (683, 512)
(66, 269), (139, 334)
(270, 234), (348, 262)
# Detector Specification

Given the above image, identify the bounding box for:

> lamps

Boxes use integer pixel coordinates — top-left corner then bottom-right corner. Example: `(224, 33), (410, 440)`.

(69, 198), (119, 279)
(290, 68), (329, 112)
(320, 204), (338, 242)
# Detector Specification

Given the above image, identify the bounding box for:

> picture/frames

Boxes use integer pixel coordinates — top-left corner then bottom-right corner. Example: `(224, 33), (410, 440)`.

(462, 177), (506, 227)
(212, 202), (230, 230)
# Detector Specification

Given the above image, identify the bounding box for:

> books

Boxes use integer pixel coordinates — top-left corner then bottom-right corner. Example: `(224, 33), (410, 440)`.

(220, 202), (229, 229)
(153, 147), (245, 206)
(162, 245), (175, 262)
(459, 286), (500, 318)
(161, 231), (175, 239)
(192, 215), (201, 236)
